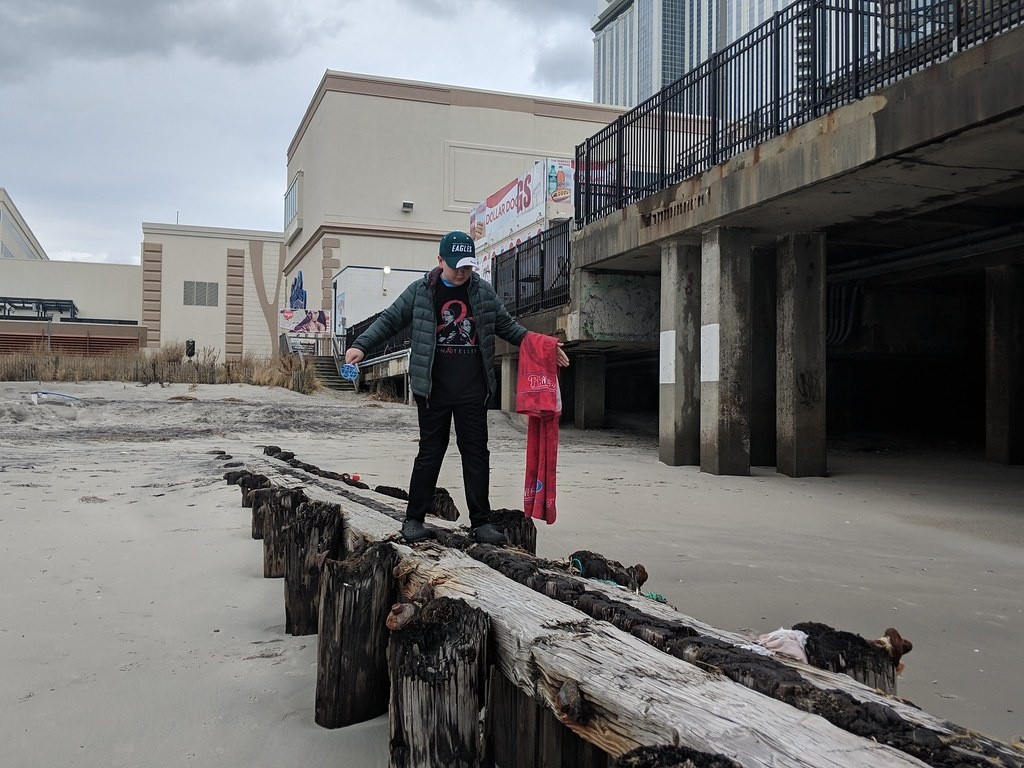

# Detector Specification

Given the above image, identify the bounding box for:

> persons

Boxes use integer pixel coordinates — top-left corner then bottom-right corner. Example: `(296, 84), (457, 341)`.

(290, 310), (326, 332)
(344, 231), (571, 544)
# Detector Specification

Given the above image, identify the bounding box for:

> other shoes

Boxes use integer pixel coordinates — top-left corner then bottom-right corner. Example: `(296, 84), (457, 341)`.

(402, 519), (427, 542)
(468, 524), (508, 544)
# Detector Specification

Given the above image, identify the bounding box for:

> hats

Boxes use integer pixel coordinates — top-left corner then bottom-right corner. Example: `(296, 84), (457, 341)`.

(440, 231), (476, 268)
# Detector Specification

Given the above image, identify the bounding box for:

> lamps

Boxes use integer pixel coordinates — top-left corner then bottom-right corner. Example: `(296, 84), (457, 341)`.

(381, 266), (391, 296)
(401, 200), (414, 213)
